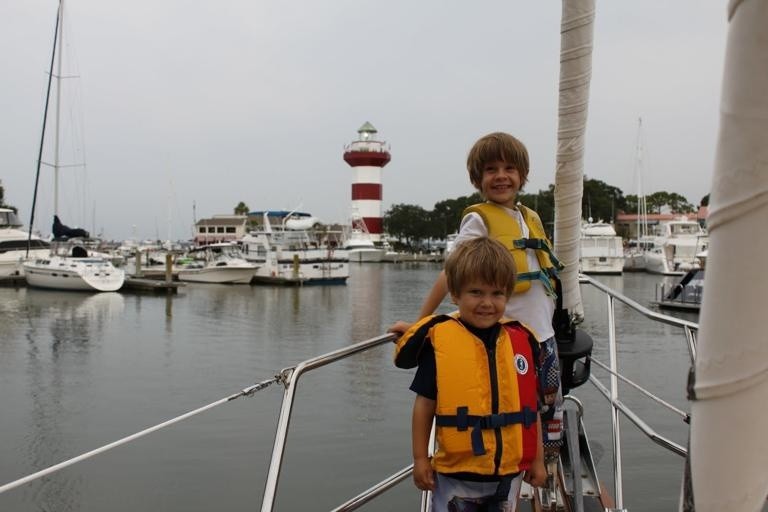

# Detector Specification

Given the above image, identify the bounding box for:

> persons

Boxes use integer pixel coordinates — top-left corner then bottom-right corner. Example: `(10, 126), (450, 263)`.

(383, 128), (567, 493)
(408, 235), (552, 510)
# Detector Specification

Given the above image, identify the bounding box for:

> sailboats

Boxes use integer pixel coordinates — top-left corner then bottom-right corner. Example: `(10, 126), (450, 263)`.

(20, 0), (125, 292)
(623, 118), (687, 275)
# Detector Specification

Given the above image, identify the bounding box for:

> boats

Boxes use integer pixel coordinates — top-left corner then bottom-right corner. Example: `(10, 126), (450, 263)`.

(628, 220), (708, 270)
(0, 178), (54, 281)
(124, 229), (351, 285)
(578, 217), (625, 273)
